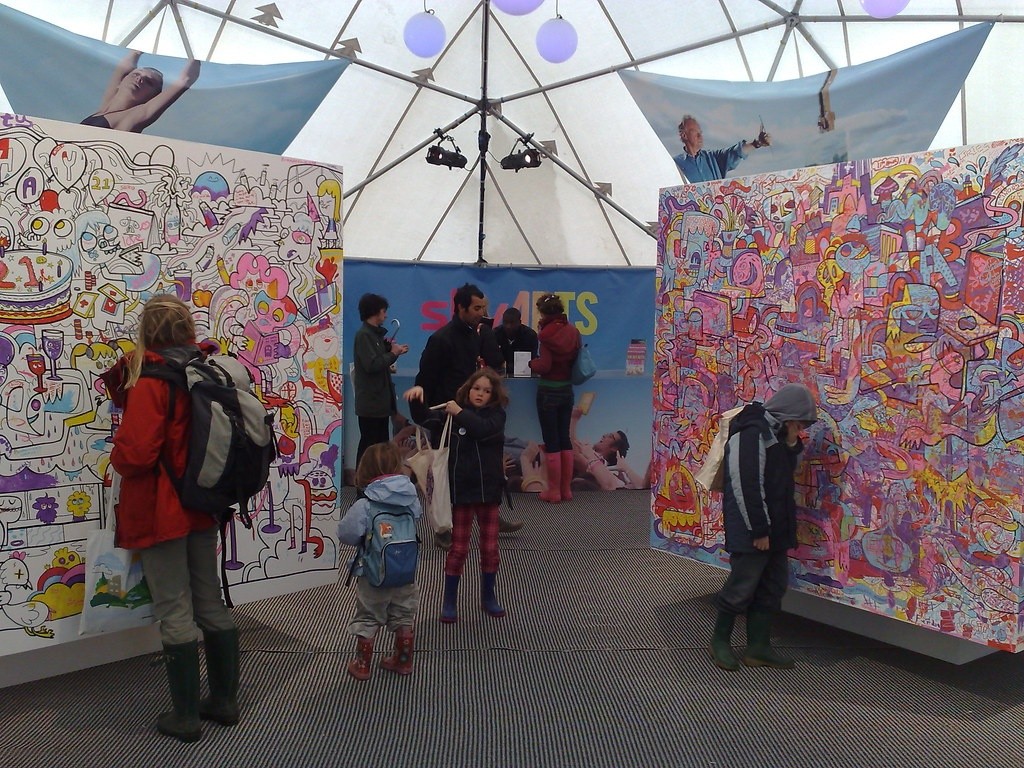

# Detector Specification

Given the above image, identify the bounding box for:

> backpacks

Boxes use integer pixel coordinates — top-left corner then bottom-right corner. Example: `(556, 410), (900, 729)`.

(357, 495), (418, 588)
(139, 352), (275, 515)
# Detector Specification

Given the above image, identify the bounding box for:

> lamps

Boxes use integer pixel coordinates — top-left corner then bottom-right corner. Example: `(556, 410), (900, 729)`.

(424, 127), (467, 171)
(499, 133), (542, 173)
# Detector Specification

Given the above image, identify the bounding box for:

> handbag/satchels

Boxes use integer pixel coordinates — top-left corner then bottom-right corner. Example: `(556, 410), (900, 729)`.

(572, 343), (596, 386)
(407, 403), (453, 533)
(695, 402), (750, 491)
(349, 361), (356, 398)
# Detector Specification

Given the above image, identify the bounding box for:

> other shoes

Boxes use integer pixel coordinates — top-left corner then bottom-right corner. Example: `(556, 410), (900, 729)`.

(497, 516), (523, 532)
(433, 529), (451, 550)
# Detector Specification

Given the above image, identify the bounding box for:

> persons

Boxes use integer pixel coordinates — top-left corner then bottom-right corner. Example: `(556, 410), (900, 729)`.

(492, 306), (538, 375)
(527, 293), (582, 502)
(707, 383), (818, 669)
(430, 366), (510, 620)
(354, 293), (409, 471)
(504, 406), (653, 491)
(335, 443), (425, 679)
(98, 293), (240, 741)
(80, 49), (201, 134)
(402, 282), (525, 550)
(674, 114), (770, 184)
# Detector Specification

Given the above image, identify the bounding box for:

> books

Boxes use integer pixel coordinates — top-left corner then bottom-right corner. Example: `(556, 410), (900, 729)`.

(578, 392), (595, 415)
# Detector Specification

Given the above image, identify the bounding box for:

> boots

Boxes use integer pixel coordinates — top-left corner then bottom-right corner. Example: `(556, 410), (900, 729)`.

(440, 573), (460, 622)
(742, 608), (794, 669)
(559, 450), (574, 500)
(538, 451), (563, 503)
(200, 625), (240, 725)
(480, 571), (506, 618)
(707, 609), (739, 671)
(381, 626), (414, 674)
(347, 634), (376, 678)
(157, 636), (202, 742)
